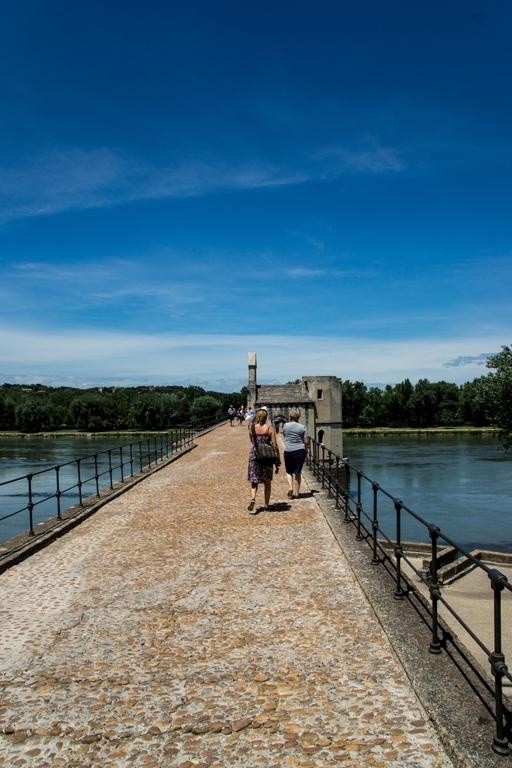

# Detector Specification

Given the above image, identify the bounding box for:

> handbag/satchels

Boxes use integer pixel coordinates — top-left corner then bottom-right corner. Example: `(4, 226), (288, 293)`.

(251, 423), (280, 465)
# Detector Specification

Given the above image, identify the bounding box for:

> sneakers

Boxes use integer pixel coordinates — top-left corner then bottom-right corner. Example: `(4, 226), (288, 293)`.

(287, 489), (299, 499)
(247, 499), (255, 511)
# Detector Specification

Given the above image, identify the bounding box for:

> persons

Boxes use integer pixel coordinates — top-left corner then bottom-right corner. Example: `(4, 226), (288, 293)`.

(281, 407), (310, 500)
(227, 402), (287, 434)
(246, 408), (282, 511)
